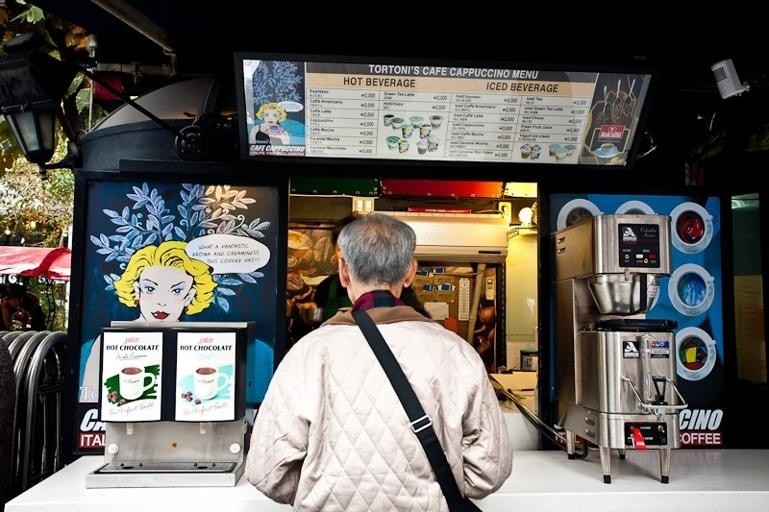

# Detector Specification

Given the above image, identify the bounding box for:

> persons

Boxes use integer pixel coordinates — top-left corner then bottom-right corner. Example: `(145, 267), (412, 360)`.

(80, 241), (219, 403)
(311, 215), (433, 322)
(249, 102), (290, 147)
(245, 214), (511, 510)
(0, 281), (46, 332)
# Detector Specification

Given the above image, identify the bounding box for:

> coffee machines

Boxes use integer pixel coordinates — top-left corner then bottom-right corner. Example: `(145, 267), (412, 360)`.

(553, 213), (689, 484)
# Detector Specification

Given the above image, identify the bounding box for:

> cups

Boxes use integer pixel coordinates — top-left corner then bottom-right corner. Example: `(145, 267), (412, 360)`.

(520, 144), (576, 161)
(383, 113), (444, 154)
(119, 366), (156, 401)
(193, 366), (229, 400)
(595, 148), (619, 164)
